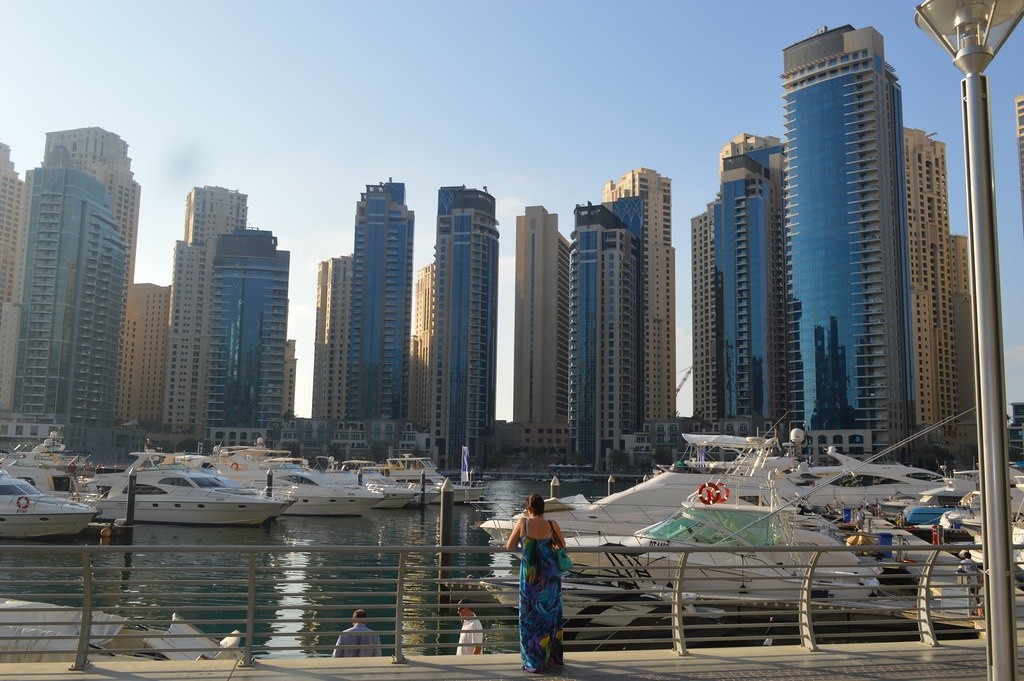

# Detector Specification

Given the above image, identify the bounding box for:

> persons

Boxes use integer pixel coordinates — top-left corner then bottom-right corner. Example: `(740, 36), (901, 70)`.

(455, 599), (484, 655)
(956, 552), (982, 615)
(856, 507), (866, 530)
(331, 609), (381, 658)
(506, 495), (567, 673)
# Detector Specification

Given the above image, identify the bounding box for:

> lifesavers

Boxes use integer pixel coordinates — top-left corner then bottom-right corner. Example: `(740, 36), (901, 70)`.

(68, 462), (75, 472)
(84, 463), (89, 467)
(932, 525), (938, 546)
(698, 482), (721, 505)
(902, 558), (918, 563)
(17, 496), (30, 509)
(97, 464), (104, 470)
(72, 493), (80, 502)
(114, 463), (121, 471)
(231, 463), (239, 470)
(715, 482), (729, 504)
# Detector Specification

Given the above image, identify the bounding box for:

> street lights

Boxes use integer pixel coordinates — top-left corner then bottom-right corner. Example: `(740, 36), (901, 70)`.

(915, 0), (1024, 681)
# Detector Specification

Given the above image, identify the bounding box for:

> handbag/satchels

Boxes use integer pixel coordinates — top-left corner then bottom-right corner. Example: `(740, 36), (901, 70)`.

(556, 548), (573, 572)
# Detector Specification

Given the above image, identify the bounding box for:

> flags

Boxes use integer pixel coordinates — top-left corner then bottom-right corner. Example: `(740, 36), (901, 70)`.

(775, 432), (785, 452)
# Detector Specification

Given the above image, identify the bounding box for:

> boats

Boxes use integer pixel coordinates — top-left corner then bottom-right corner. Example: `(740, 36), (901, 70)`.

(481, 433), (1024, 623)
(0, 431), (480, 538)
(0, 596), (245, 660)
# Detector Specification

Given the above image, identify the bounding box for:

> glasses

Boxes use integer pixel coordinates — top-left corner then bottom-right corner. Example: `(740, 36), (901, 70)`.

(457, 609), (461, 612)
